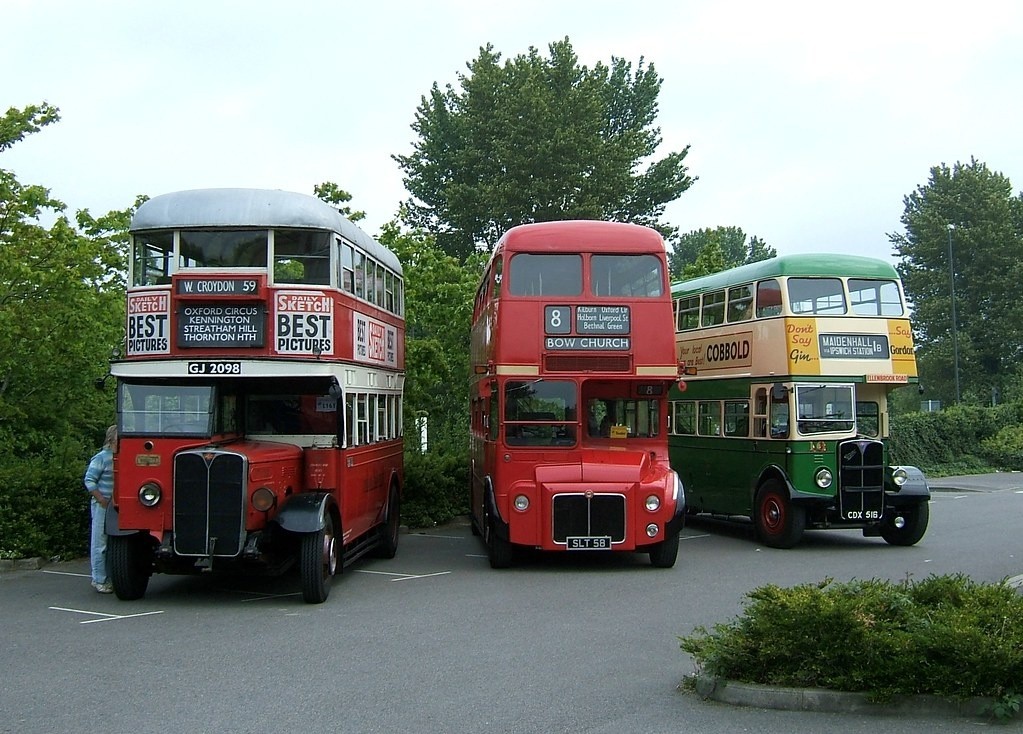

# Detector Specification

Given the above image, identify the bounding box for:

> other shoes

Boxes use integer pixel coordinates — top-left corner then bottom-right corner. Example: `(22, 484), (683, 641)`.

(90, 578), (114, 594)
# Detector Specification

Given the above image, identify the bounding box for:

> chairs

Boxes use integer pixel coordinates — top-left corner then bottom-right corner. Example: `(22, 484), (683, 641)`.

(163, 421), (207, 432)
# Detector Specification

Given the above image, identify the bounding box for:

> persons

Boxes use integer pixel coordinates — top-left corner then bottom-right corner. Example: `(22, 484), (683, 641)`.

(84, 424), (118, 594)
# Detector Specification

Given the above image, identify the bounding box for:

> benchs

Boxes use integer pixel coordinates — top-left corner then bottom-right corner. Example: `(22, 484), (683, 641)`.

(517, 411), (557, 437)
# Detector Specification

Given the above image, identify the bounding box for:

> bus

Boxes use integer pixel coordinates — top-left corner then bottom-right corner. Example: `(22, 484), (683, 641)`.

(103, 189), (407, 605)
(619, 253), (931, 546)
(468, 218), (688, 572)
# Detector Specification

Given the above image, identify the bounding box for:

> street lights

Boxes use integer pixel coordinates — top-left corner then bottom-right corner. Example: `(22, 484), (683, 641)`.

(941, 224), (960, 405)
